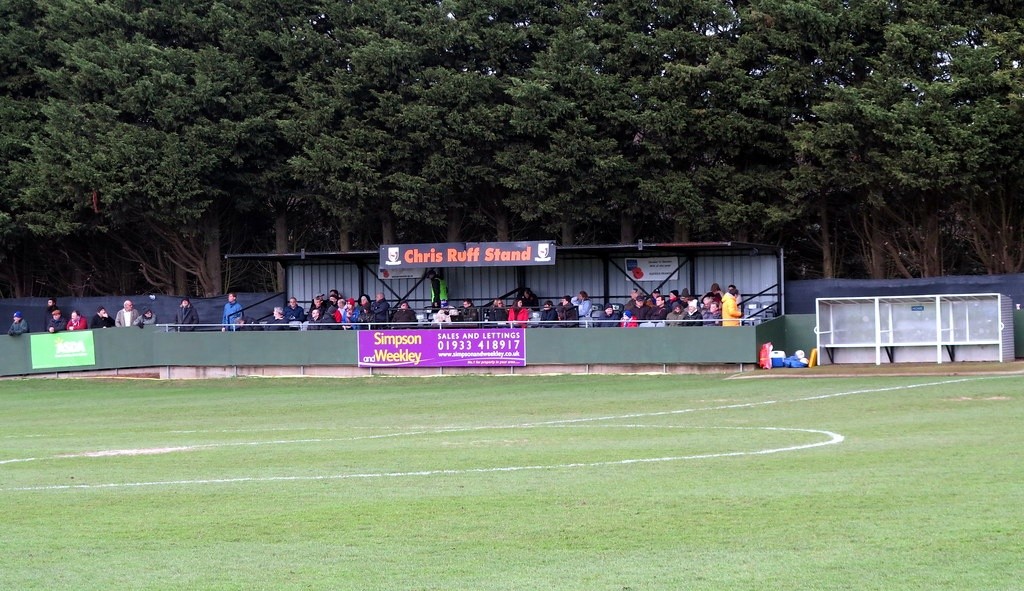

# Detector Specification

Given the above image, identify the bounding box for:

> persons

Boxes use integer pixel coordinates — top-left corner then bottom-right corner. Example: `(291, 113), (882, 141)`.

(596, 284), (742, 328)
(8, 310), (30, 335)
(221, 293), (264, 331)
(47, 309), (68, 332)
(44, 298), (59, 331)
(174, 297), (199, 332)
(66, 310), (88, 330)
(91, 306), (115, 328)
(426, 271), (592, 328)
(115, 300), (139, 327)
(133, 307), (156, 329)
(265, 289), (416, 331)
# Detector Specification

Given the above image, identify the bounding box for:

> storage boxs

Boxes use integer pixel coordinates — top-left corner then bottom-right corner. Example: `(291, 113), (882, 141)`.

(771, 351), (786, 367)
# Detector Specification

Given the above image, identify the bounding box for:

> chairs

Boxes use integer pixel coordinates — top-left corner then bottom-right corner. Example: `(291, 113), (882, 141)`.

(421, 301), (777, 326)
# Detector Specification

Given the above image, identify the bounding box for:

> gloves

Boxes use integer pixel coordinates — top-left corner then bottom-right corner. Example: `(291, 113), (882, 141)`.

(138, 321), (145, 329)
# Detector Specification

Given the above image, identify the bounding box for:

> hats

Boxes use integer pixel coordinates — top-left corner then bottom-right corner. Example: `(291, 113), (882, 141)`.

(710, 283), (721, 291)
(345, 297), (355, 306)
(688, 299), (698, 309)
(653, 288), (660, 294)
(181, 297), (190, 303)
(51, 310), (60, 314)
(400, 300), (407, 304)
(682, 288), (690, 297)
(97, 306), (104, 313)
(671, 290), (679, 298)
(636, 295), (644, 302)
(142, 306), (152, 315)
(624, 310), (632, 318)
(14, 311), (22, 318)
(604, 303), (613, 310)
(427, 270), (436, 279)
(274, 306), (284, 314)
